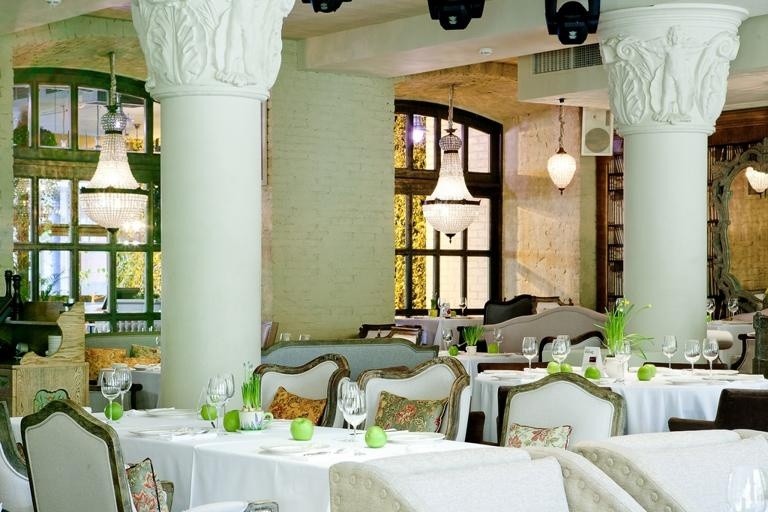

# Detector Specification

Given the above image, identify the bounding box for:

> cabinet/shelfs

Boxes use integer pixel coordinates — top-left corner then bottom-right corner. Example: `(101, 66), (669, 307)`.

(596, 129), (768, 320)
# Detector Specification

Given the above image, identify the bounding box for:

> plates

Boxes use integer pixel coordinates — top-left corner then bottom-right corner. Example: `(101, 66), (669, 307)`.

(384, 428), (446, 443)
(482, 353), (513, 357)
(130, 404), (202, 442)
(482, 368), (524, 383)
(258, 443), (327, 458)
(731, 322), (754, 326)
(707, 369), (741, 382)
(135, 362), (160, 371)
(411, 315), (426, 318)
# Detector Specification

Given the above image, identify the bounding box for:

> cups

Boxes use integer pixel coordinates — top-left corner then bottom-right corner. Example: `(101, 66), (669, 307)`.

(280, 333), (311, 342)
(48, 335), (62, 355)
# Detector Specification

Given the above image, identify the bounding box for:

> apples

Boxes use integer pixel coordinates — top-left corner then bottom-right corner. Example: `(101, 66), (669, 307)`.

(548, 362), (560, 374)
(366, 425), (386, 448)
(449, 345), (457, 356)
(104, 403), (123, 420)
(429, 310), (437, 317)
(451, 310), (455, 316)
(290, 418), (313, 441)
(223, 410), (240, 432)
(562, 364), (571, 372)
(585, 366), (600, 379)
(638, 364), (655, 380)
(488, 342), (497, 352)
(201, 405), (217, 420)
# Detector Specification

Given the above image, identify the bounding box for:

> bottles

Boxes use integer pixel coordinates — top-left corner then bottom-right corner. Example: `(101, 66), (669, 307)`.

(580, 346), (604, 379)
(4, 268), (24, 322)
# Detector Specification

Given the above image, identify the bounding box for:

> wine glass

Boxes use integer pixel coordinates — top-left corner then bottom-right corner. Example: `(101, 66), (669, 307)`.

(522, 336), (538, 369)
(441, 327), (455, 356)
(706, 297), (716, 320)
(98, 363), (131, 430)
(438, 297), (466, 319)
(726, 297), (738, 320)
(338, 379), (366, 449)
(552, 334), (571, 371)
(660, 334), (719, 375)
(197, 372), (237, 437)
(493, 328), (504, 353)
(605, 338), (631, 379)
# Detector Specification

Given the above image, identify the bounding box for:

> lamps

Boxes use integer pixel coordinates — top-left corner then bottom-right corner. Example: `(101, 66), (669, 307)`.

(745, 167), (768, 194)
(546, 97), (577, 195)
(422, 83), (481, 244)
(302, 0), (351, 13)
(544, 0), (600, 45)
(78, 46), (150, 235)
(427, 0), (485, 30)
